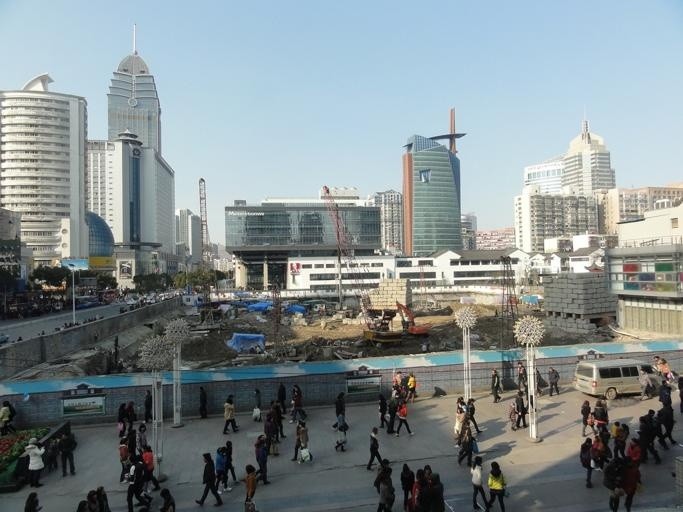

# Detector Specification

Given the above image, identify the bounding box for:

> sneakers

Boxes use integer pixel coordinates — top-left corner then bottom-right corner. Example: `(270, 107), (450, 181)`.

(152, 486), (161, 491)
(223, 428), (229, 434)
(288, 419), (297, 424)
(224, 487), (231, 492)
(120, 480), (127, 484)
(30, 483), (44, 488)
(263, 482), (270, 485)
(291, 458), (297, 461)
(232, 426), (238, 432)
(477, 431), (483, 434)
(394, 434), (400, 439)
(410, 432), (415, 436)
(216, 490), (222, 495)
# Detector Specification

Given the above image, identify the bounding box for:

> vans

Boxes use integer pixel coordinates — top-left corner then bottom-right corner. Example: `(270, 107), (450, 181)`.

(572, 360), (667, 400)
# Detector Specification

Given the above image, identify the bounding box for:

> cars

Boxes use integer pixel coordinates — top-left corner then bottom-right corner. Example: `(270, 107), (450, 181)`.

(0, 332), (8, 343)
(252, 293), (270, 298)
(121, 285), (209, 307)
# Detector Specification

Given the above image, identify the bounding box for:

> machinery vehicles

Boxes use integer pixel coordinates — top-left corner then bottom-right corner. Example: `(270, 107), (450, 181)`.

(322, 185), (401, 349)
(392, 301), (432, 337)
(318, 302), (338, 317)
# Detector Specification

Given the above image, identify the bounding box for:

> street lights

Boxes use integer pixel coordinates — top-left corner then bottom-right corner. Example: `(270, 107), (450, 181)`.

(138, 332), (172, 485)
(67, 262), (76, 326)
(453, 305), (478, 401)
(164, 316), (190, 427)
(512, 315), (546, 442)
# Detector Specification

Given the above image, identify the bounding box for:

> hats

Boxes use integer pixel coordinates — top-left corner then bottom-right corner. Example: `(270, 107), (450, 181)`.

(29, 438), (38, 444)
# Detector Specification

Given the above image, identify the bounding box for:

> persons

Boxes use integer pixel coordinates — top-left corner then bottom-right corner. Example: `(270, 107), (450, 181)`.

(24, 437), (45, 487)
(118, 359), (144, 372)
(374, 459), (446, 512)
(75, 500), (90, 512)
(115, 390), (176, 512)
(453, 396), (507, 512)
(0, 400), (17, 434)
(46, 436), (60, 472)
(490, 361), (560, 431)
(95, 486), (110, 511)
(23, 491), (41, 511)
(195, 383), (313, 511)
(86, 489), (100, 511)
(333, 391), (349, 453)
(581, 357), (683, 512)
(0, 287), (145, 320)
(57, 430), (77, 477)
(367, 371), (416, 468)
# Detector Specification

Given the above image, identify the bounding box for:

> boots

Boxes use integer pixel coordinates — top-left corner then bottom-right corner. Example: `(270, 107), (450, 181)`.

(334, 443), (340, 452)
(341, 445), (346, 452)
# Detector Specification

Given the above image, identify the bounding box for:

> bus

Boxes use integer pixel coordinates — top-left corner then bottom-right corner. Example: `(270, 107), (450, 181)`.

(75, 289), (121, 309)
(233, 290), (248, 297)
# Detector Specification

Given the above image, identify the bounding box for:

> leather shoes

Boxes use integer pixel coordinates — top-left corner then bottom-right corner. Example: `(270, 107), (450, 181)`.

(195, 500), (203, 505)
(214, 501), (223, 507)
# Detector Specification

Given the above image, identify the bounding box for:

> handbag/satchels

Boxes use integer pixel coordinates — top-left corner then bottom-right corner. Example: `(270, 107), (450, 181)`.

(253, 407), (260, 420)
(300, 447), (313, 462)
(501, 474), (511, 498)
(245, 498), (256, 512)
(614, 486), (626, 496)
(590, 457), (602, 470)
(118, 422), (123, 431)
